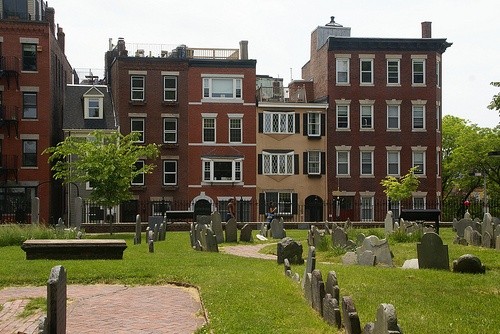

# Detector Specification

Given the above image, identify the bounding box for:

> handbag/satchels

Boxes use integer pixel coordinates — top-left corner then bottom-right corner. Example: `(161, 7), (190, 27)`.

(267, 213), (273, 219)
(226, 212), (232, 217)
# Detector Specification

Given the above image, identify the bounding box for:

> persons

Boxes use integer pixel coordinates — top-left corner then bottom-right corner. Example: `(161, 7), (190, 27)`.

(227, 198), (236, 222)
(267, 201), (277, 230)
